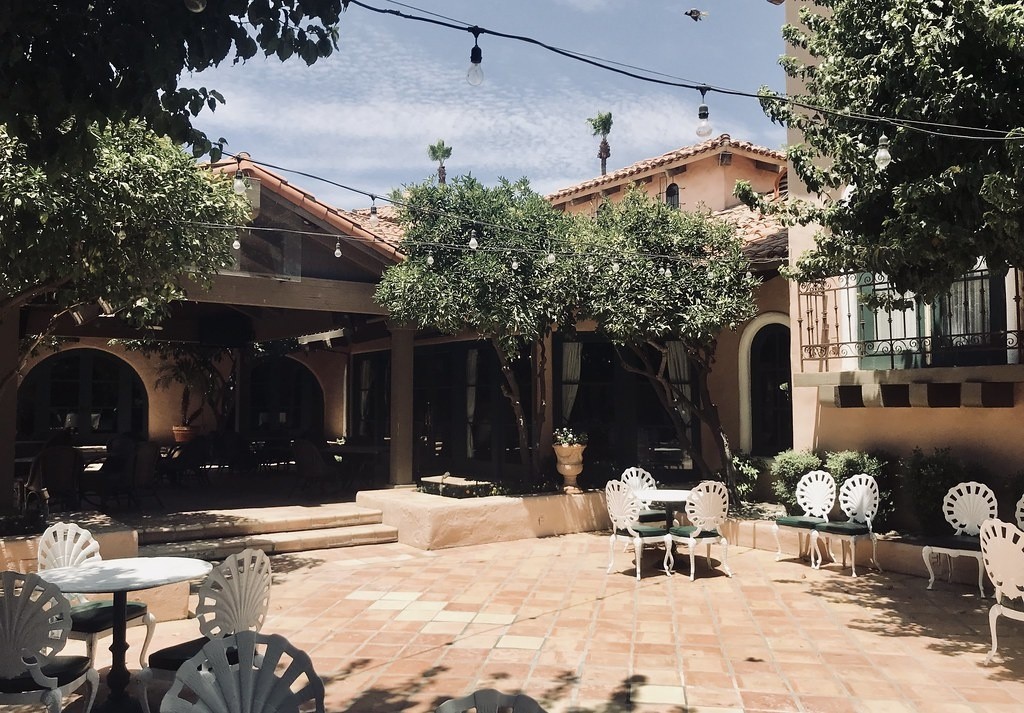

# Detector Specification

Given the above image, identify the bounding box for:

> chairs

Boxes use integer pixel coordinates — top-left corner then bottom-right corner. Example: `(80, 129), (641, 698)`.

(667, 481), (731, 581)
(620, 467), (679, 553)
(159, 630), (325, 713)
(921, 482), (998, 598)
(605, 479), (672, 581)
(149, 547), (272, 671)
(979, 518), (1024, 656)
(0, 571), (100, 713)
(1015, 494), (1024, 531)
(772, 469), (837, 569)
(811, 473), (884, 578)
(13, 429), (376, 512)
(38, 522), (157, 668)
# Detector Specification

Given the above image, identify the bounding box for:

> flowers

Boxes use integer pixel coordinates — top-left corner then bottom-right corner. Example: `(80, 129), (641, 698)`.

(551, 426), (588, 445)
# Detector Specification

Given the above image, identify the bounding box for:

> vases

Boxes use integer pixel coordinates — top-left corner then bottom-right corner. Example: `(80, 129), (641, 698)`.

(552, 446), (586, 493)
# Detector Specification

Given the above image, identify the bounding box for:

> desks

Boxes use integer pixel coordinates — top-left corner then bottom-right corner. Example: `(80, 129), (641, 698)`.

(318, 448), (381, 468)
(622, 490), (702, 570)
(22, 557), (214, 713)
(14, 451), (110, 507)
